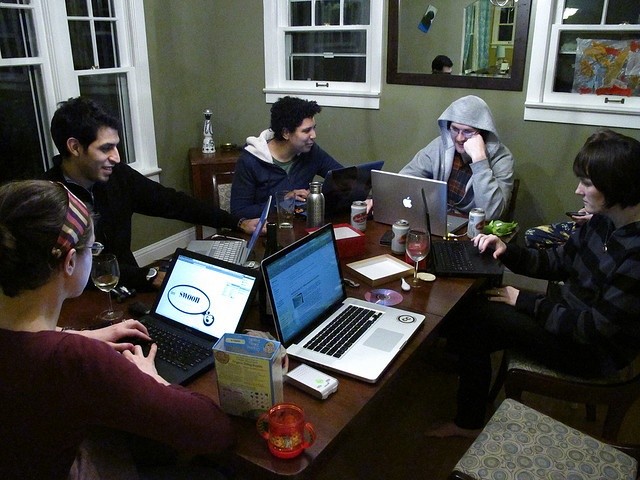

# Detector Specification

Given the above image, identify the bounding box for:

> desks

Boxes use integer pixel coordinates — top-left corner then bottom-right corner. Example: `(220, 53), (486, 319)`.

(58, 196), (521, 480)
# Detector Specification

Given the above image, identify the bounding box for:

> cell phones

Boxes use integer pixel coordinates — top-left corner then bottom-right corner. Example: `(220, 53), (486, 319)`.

(565, 210), (586, 217)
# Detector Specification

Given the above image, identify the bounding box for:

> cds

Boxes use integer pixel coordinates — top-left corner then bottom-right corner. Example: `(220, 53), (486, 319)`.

(363, 287), (403, 306)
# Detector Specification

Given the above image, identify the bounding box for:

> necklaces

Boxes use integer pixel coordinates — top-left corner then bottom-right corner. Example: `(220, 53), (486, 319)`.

(602, 225), (616, 253)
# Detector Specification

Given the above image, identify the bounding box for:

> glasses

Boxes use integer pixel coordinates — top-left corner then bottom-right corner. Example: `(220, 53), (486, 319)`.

(448, 126), (476, 139)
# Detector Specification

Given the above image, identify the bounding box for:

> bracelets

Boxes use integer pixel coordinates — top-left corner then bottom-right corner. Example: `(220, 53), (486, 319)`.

(238, 218), (248, 229)
(59, 326), (75, 333)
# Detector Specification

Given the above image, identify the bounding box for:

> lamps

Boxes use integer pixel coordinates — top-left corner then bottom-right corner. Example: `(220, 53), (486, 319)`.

(496, 45), (506, 63)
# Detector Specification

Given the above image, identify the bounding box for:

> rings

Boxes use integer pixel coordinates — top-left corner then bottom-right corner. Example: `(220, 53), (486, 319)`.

(497, 290), (500, 297)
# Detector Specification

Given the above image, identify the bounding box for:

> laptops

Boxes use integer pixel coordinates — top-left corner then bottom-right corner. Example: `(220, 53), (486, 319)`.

(370, 167), (471, 236)
(183, 193), (273, 267)
(421, 186), (506, 281)
(262, 222), (426, 385)
(292, 159), (386, 216)
(126, 245), (258, 392)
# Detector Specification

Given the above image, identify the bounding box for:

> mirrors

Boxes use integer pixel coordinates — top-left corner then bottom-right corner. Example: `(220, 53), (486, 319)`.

(385, 0), (533, 93)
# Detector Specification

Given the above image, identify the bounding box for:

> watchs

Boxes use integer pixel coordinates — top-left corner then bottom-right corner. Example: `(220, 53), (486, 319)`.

(146, 268), (158, 287)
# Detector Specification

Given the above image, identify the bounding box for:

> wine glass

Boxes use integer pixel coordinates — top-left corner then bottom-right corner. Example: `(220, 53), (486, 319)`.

(405, 228), (431, 287)
(91, 254), (124, 321)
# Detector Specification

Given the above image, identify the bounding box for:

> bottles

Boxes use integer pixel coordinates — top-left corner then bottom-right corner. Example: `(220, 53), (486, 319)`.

(307, 182), (326, 230)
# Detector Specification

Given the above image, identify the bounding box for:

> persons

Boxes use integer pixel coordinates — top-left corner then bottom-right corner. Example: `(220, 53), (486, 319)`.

(424, 139), (639, 439)
(41, 96), (268, 295)
(432, 55), (453, 74)
(399, 95), (514, 222)
(524, 130), (616, 251)
(230, 97), (344, 218)
(0, 180), (230, 480)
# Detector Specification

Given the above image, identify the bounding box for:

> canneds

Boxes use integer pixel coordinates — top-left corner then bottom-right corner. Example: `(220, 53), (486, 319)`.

(392, 219), (409, 254)
(466, 208), (486, 239)
(351, 201), (367, 230)
(244, 262), (261, 304)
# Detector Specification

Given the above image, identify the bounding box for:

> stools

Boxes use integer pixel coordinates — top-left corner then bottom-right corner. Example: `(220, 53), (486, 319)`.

(451, 396), (640, 479)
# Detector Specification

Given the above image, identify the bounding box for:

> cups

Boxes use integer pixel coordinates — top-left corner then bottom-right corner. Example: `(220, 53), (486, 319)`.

(257, 404), (315, 459)
(275, 190), (296, 230)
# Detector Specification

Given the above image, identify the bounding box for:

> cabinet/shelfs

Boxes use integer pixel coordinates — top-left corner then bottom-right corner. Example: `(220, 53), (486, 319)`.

(261, 0), (390, 110)
(523, 0), (640, 131)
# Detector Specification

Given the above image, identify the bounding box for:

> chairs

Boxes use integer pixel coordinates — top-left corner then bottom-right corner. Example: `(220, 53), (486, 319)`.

(210, 168), (237, 234)
(498, 176), (519, 223)
(490, 278), (639, 444)
